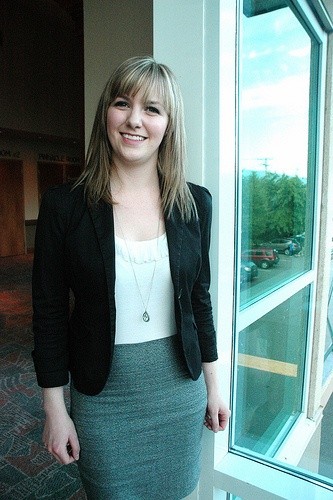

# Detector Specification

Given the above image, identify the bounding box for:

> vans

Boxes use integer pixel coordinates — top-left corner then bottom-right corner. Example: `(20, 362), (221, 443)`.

(241, 258), (258, 281)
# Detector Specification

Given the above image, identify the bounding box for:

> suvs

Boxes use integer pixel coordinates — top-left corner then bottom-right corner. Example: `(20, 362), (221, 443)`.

(259, 238), (302, 256)
(243, 248), (279, 269)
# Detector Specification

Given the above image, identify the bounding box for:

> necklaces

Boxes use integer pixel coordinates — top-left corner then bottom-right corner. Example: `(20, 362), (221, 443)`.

(107, 182), (162, 323)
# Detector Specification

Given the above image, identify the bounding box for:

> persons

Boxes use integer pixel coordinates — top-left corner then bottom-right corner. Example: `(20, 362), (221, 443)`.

(31, 56), (234, 500)
